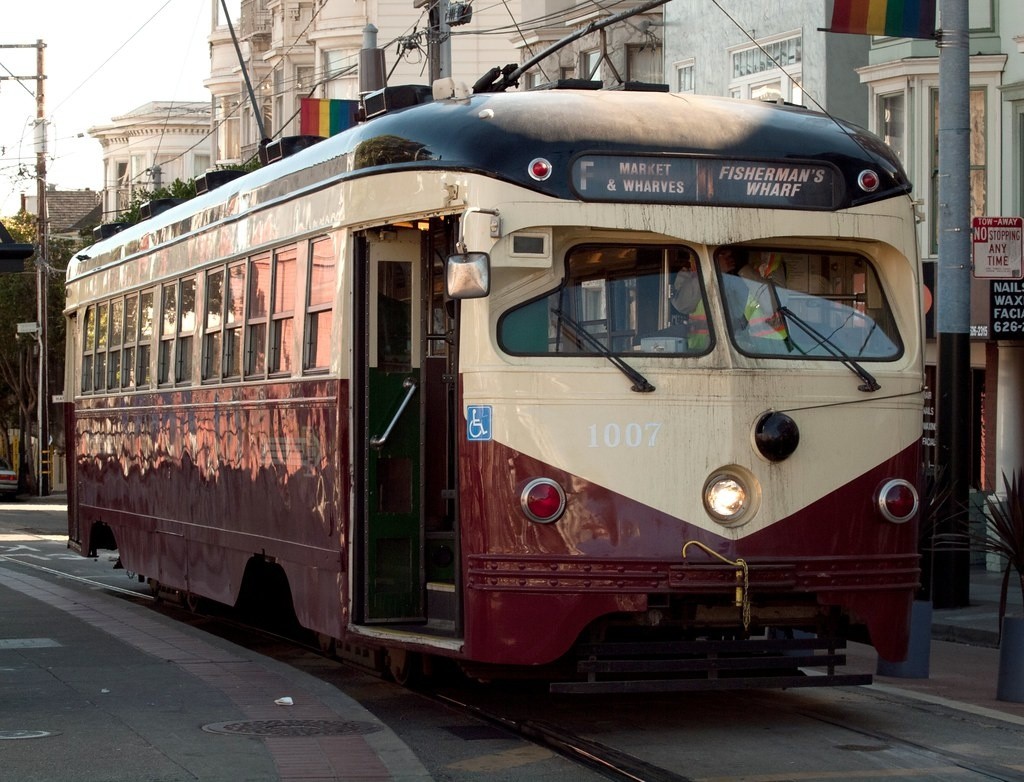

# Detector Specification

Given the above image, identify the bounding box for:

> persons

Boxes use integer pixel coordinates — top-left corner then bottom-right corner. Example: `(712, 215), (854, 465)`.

(668, 247), (789, 354)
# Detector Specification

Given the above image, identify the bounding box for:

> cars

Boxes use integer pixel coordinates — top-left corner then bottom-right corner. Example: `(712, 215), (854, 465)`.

(0, 457), (19, 501)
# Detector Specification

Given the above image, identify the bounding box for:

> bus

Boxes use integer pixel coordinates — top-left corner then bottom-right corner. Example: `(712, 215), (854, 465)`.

(60, 0), (925, 706)
